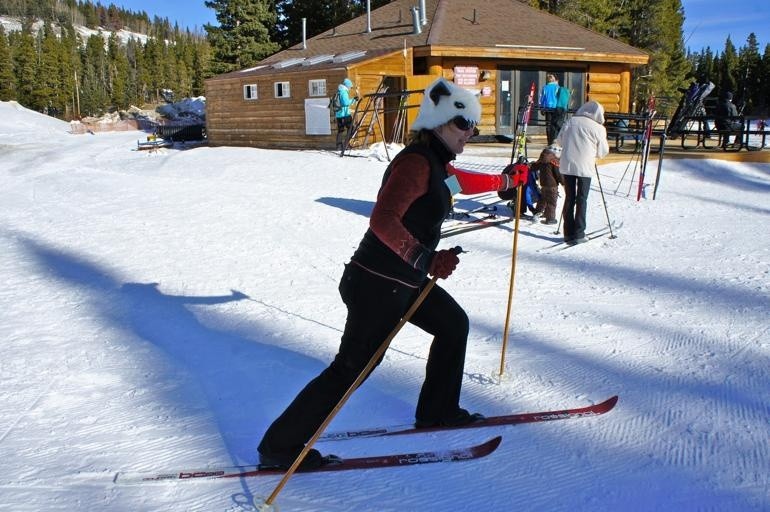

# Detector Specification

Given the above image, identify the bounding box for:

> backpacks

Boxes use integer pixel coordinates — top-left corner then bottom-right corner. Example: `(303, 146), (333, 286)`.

(556, 85), (571, 112)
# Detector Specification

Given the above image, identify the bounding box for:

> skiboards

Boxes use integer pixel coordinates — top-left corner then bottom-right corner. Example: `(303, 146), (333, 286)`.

(389, 89), (409, 144)
(537, 221), (624, 254)
(114, 394), (618, 485)
(439, 216), (513, 238)
(349, 83), (389, 149)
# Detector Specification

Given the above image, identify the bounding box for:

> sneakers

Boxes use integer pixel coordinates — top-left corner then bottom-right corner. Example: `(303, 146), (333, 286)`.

(511, 214), (558, 224)
(566, 237), (590, 244)
(720, 141), (743, 148)
(258, 449), (343, 472)
(414, 409), (484, 428)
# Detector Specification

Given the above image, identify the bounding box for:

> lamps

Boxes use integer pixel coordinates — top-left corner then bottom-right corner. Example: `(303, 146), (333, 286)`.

(480, 69), (491, 81)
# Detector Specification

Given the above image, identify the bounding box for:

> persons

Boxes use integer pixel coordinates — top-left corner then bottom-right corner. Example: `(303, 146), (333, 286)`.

(256, 75), (533, 468)
(530, 138), (566, 224)
(334, 78), (359, 150)
(497, 162), (541, 220)
(541, 70), (562, 146)
(685, 80), (712, 138)
(715, 91), (746, 147)
(557, 101), (610, 244)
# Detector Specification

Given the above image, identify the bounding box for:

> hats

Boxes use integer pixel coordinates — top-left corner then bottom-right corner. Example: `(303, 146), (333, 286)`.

(343, 78), (353, 89)
(409, 77), (482, 130)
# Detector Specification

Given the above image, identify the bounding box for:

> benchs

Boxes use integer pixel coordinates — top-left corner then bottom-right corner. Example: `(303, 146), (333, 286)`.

(603, 112), (770, 154)
